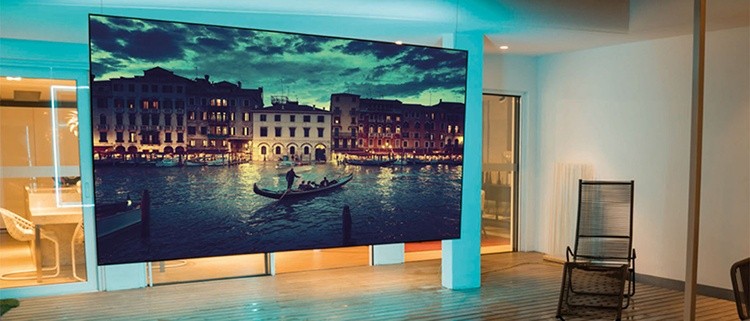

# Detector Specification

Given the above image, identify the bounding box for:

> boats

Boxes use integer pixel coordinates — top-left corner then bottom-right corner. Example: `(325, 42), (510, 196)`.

(253, 171), (354, 201)
(95, 185), (151, 255)
(94, 158), (245, 168)
(348, 158), (463, 166)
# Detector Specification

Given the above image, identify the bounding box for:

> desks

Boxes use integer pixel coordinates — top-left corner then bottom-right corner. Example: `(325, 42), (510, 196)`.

(28, 188), (84, 283)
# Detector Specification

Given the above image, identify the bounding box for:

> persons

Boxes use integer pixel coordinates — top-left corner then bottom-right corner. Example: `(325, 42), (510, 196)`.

(286, 168), (300, 189)
(298, 177), (328, 191)
(291, 154), (298, 161)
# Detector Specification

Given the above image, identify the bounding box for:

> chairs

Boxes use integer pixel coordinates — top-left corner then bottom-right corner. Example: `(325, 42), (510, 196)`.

(0, 207), (87, 283)
(555, 180), (636, 321)
(730, 257), (750, 321)
